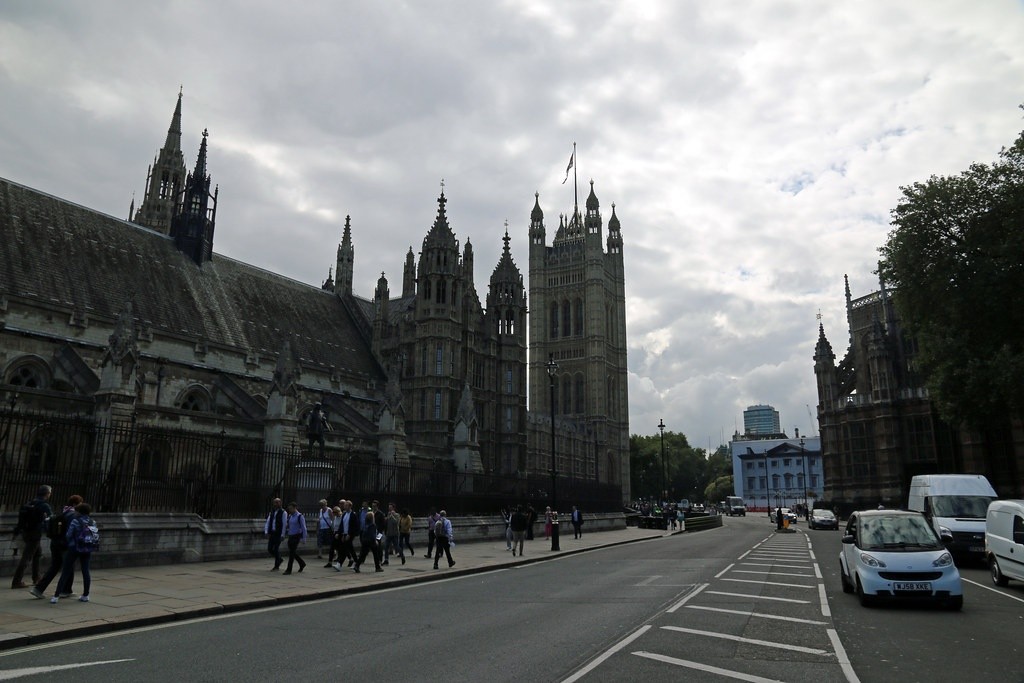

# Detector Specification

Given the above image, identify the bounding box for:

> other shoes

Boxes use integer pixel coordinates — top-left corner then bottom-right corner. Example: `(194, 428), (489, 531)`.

(51, 596), (58, 603)
(519, 549), (522, 556)
(12, 582), (29, 588)
(512, 549), (515, 556)
(382, 561), (389, 566)
(271, 558), (285, 571)
(353, 566), (360, 573)
(30, 588), (46, 599)
(375, 568), (384, 572)
(324, 563), (333, 568)
(79, 596), (91, 602)
(59, 592), (77, 598)
(449, 561), (456, 567)
(282, 570), (291, 575)
(298, 563), (306, 572)
(424, 554), (432, 558)
(401, 556), (406, 565)
(433, 563), (439, 569)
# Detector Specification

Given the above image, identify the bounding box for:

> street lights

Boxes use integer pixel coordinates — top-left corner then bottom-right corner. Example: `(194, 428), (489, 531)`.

(658, 418), (666, 502)
(545, 357), (560, 551)
(763, 449), (770, 516)
(665, 443), (670, 502)
(799, 439), (809, 520)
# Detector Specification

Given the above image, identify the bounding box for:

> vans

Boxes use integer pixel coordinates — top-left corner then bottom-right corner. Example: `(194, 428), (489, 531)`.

(725, 496), (747, 516)
(907, 474), (1000, 568)
(985, 499), (1024, 587)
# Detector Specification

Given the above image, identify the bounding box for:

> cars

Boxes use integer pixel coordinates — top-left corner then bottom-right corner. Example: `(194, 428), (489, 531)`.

(808, 509), (838, 530)
(838, 506), (963, 609)
(770, 508), (797, 524)
(718, 500), (725, 514)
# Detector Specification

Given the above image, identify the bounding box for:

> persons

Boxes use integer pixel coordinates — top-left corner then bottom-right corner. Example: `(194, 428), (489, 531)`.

(572, 506), (582, 539)
(305, 402), (331, 459)
(316, 498), (414, 573)
(669, 508), (684, 530)
(424, 510), (455, 569)
(545, 505), (552, 540)
(11, 485), (97, 603)
(500, 502), (538, 556)
(777, 507), (782, 529)
(265, 497), (307, 575)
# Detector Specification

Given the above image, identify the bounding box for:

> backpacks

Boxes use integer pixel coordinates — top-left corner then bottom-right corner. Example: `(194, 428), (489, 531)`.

(435, 519), (446, 537)
(75, 518), (101, 553)
(43, 511), (71, 535)
